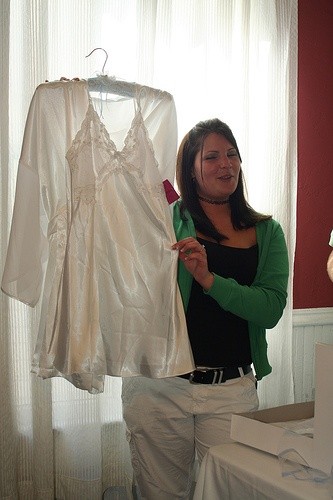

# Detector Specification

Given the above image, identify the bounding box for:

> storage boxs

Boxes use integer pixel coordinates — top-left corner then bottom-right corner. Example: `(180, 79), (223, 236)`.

(228, 343), (333, 476)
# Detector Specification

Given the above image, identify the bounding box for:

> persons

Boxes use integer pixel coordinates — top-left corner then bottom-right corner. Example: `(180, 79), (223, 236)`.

(42, 77), (293, 500)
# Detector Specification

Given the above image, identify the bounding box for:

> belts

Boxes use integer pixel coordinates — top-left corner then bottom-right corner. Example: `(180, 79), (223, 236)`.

(174, 364), (252, 384)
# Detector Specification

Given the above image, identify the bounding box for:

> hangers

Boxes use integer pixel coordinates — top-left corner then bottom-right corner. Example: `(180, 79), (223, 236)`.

(35, 47), (175, 108)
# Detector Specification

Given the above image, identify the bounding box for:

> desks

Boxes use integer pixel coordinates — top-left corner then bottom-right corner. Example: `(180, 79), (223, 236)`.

(206, 441), (333, 500)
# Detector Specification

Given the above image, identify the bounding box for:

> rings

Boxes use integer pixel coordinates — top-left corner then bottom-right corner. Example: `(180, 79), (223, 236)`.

(200, 243), (205, 250)
